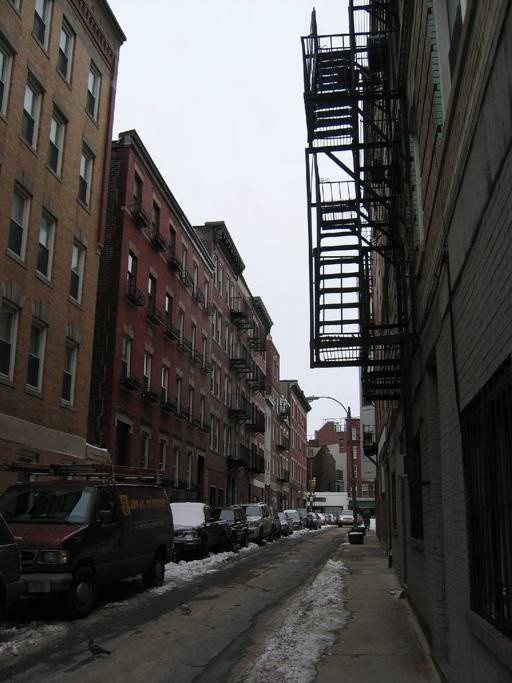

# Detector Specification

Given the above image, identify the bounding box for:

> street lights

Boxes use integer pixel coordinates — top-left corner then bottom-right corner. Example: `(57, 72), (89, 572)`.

(304, 392), (359, 529)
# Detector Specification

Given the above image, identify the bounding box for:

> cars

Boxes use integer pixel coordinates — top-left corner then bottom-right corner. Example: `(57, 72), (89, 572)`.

(337, 508), (362, 527)
(0, 511), (22, 623)
(170, 501), (335, 561)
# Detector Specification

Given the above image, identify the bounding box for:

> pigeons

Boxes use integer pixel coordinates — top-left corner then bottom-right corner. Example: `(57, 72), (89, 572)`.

(179, 600), (193, 615)
(88, 638), (112, 658)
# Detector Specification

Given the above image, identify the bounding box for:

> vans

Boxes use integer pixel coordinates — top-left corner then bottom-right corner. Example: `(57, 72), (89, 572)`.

(1, 461), (175, 617)
